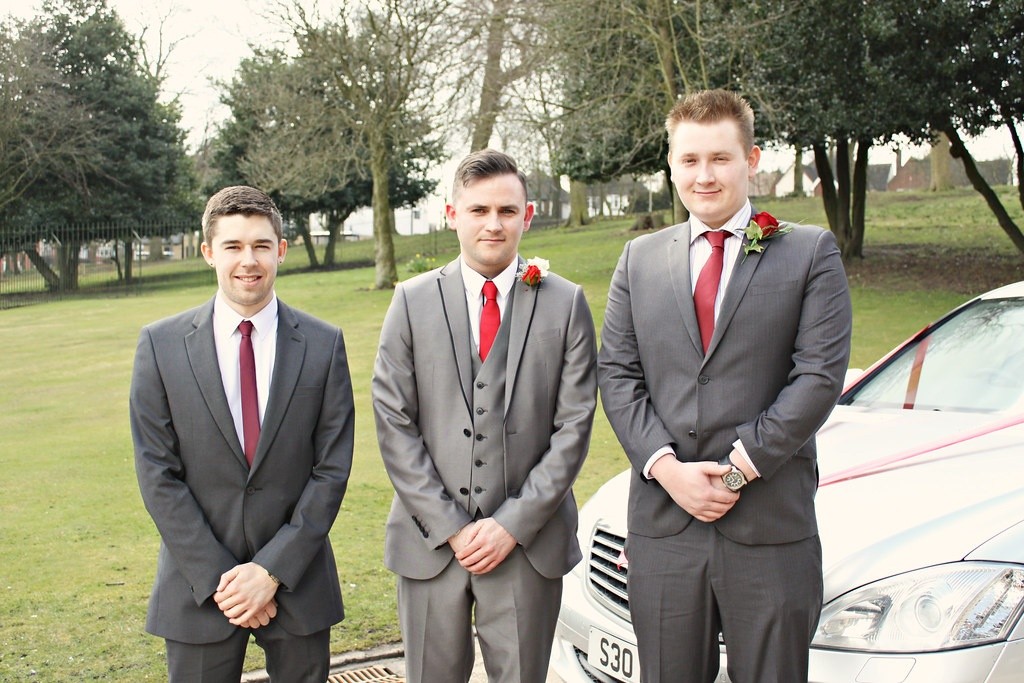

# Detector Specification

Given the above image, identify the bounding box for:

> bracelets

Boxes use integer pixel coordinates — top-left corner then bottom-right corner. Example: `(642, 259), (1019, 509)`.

(268, 573), (280, 584)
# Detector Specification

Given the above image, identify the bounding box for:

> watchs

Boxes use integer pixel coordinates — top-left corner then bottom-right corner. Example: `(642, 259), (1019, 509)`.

(718, 455), (748, 493)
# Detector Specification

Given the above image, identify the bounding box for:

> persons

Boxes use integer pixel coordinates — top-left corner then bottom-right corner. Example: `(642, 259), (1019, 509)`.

(598, 88), (853, 683)
(371, 150), (597, 683)
(129, 186), (355, 683)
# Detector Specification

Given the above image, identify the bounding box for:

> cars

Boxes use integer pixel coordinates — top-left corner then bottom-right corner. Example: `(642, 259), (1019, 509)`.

(555, 277), (1024, 683)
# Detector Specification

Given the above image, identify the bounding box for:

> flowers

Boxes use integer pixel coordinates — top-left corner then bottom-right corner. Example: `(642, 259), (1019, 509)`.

(516, 256), (550, 287)
(735, 213), (792, 265)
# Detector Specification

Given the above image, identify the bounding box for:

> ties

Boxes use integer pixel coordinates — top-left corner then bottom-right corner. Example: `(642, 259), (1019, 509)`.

(238, 322), (261, 468)
(693, 231), (734, 357)
(479, 281), (501, 362)
